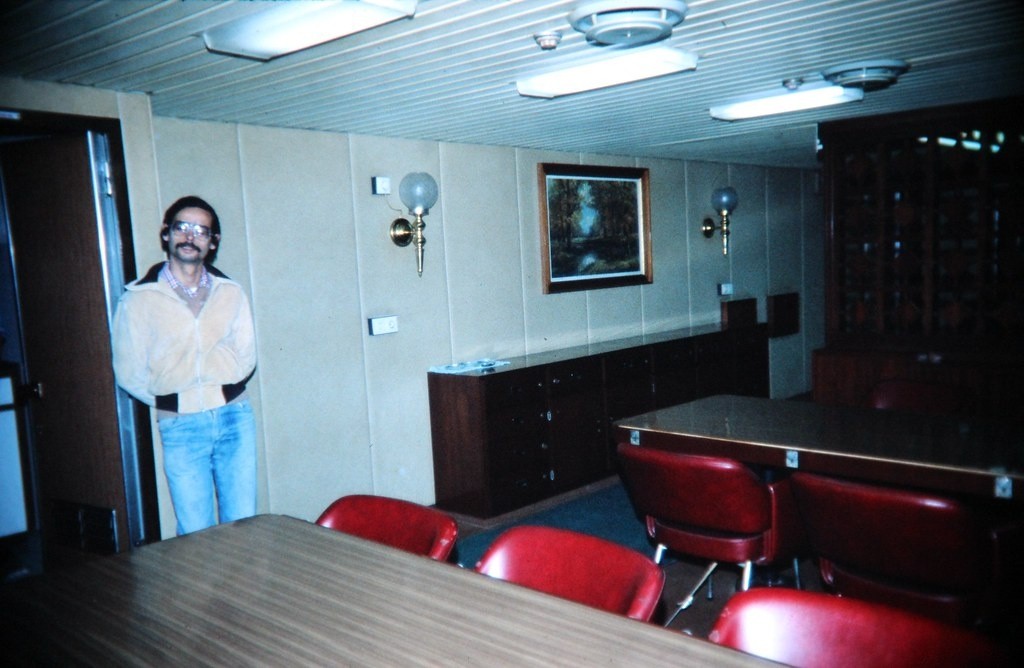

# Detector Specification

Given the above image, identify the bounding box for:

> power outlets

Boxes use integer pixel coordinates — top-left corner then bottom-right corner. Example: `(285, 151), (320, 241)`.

(371, 175), (391, 195)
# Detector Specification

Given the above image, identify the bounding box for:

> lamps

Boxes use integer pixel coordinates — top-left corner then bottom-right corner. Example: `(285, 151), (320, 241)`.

(702, 187), (737, 256)
(204, 0), (418, 60)
(390, 171), (435, 276)
(711, 88), (865, 122)
(516, 47), (701, 99)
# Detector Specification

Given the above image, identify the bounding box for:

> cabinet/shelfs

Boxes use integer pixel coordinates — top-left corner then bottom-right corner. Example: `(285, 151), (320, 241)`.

(427, 322), (771, 523)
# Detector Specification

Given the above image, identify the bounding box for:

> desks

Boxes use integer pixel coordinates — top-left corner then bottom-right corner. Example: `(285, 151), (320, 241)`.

(616, 392), (1024, 492)
(1, 513), (795, 668)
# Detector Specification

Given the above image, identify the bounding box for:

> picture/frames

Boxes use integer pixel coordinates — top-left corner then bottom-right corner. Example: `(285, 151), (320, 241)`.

(537, 164), (654, 292)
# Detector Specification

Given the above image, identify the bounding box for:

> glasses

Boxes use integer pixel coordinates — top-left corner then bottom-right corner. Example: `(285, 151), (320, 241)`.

(172, 221), (212, 241)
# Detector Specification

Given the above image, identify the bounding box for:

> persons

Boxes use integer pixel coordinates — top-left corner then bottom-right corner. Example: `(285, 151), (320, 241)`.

(110, 195), (258, 537)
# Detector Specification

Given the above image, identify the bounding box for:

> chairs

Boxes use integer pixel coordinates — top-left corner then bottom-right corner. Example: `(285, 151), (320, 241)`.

(710, 588), (1018, 668)
(617, 442), (806, 591)
(861, 379), (970, 416)
(791, 472), (1002, 623)
(313, 495), (457, 561)
(473, 526), (667, 621)
